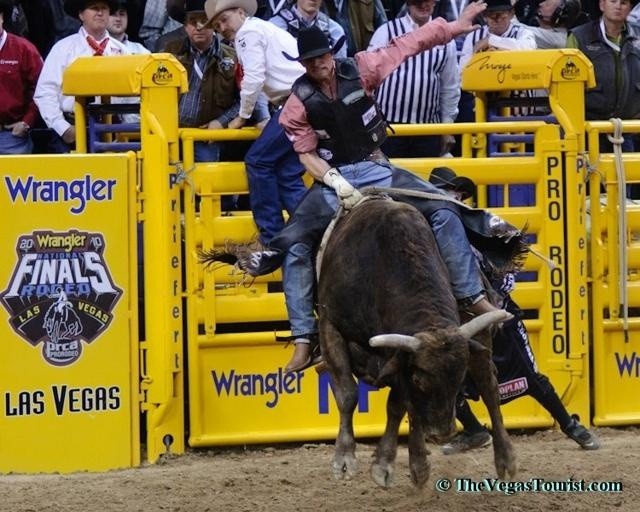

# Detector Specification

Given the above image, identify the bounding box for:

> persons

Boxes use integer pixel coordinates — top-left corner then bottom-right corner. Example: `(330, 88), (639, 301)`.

(198, 0), (312, 249)
(0, 0), (640, 162)
(428, 167), (600, 454)
(278, 1), (515, 372)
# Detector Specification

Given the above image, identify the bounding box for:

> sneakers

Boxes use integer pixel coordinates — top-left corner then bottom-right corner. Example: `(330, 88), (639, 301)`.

(561, 414), (600, 449)
(440, 427), (492, 455)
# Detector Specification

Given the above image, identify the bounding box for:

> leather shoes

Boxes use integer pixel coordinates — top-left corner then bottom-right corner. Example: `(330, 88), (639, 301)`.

(285, 335), (318, 372)
(466, 298), (514, 323)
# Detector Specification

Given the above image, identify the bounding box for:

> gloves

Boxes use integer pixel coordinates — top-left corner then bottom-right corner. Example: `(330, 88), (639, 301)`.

(322, 167), (363, 208)
(500, 272), (515, 294)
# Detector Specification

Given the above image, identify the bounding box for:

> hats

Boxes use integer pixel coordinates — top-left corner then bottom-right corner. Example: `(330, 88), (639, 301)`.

(63, 1), (582, 61)
(429, 167), (476, 199)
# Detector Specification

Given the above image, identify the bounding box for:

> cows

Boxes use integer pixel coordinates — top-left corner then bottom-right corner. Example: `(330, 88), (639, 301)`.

(317, 198), (518, 491)
